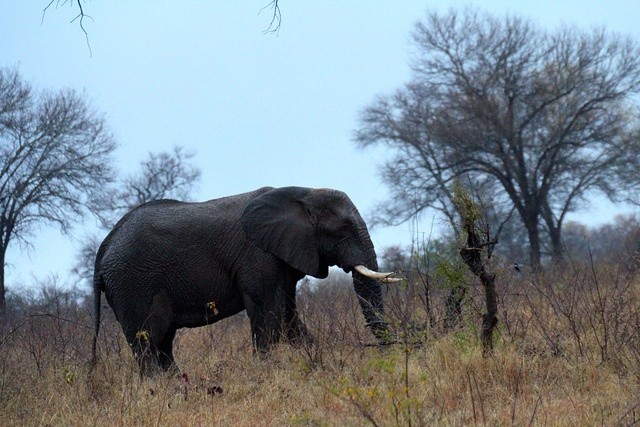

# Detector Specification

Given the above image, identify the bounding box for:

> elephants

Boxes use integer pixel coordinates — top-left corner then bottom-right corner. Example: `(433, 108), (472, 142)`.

(88, 185), (406, 380)
